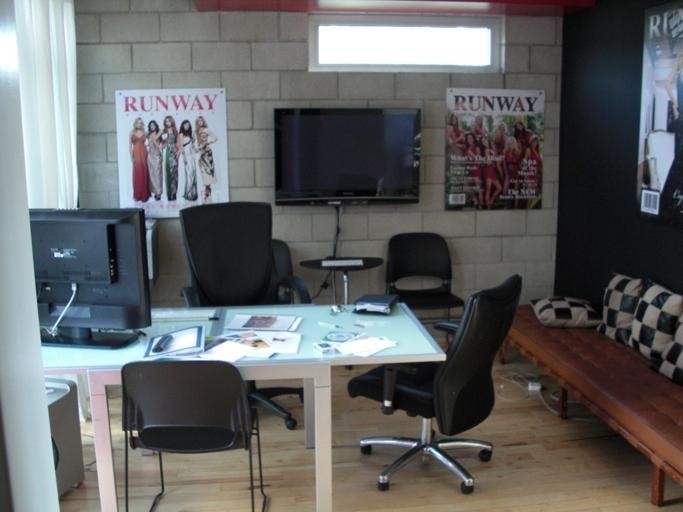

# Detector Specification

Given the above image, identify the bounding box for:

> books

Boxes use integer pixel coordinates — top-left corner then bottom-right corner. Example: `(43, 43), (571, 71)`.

(143, 326), (204, 359)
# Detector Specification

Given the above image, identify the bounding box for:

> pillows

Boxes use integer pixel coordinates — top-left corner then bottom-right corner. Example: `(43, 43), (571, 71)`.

(596, 271), (643, 346)
(529, 296), (603, 328)
(628, 277), (683, 387)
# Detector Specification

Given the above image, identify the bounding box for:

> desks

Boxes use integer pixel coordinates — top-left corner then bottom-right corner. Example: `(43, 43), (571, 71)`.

(37, 302), (448, 512)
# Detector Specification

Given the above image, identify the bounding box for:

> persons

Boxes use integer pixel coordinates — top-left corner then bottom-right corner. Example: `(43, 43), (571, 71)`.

(194, 116), (217, 199)
(163, 115), (180, 201)
(146, 120), (163, 201)
(179, 120), (198, 201)
(448, 114), (542, 209)
(129, 117), (151, 202)
(659, 49), (683, 215)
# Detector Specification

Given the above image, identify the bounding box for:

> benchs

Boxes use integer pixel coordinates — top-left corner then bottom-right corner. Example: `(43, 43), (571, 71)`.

(504, 304), (683, 507)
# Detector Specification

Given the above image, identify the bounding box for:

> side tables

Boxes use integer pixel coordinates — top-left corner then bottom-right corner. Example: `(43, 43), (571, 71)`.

(299, 256), (384, 304)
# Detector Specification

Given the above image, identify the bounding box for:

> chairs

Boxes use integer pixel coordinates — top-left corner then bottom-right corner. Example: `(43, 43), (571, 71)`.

(121, 360), (267, 512)
(386, 232), (465, 344)
(348, 273), (522, 495)
(178, 200), (312, 430)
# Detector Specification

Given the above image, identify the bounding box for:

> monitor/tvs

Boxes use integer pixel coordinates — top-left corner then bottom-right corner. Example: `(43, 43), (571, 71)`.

(274, 107), (421, 206)
(29, 208), (151, 349)
